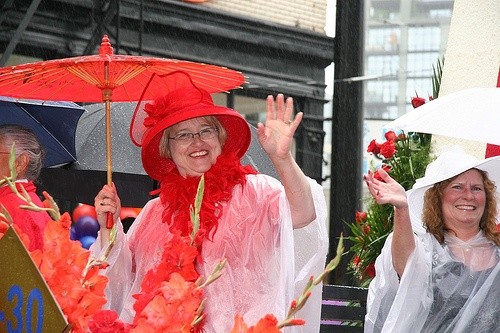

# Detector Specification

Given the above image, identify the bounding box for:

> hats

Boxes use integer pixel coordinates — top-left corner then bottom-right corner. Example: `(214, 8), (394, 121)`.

(141, 85), (251, 179)
(405, 151), (500, 232)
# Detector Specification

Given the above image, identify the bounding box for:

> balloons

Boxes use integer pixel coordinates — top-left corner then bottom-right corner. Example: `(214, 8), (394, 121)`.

(70, 202), (136, 250)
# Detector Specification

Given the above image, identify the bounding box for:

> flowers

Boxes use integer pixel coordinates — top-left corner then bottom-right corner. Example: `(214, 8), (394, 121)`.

(335, 56), (445, 333)
(0, 140), (344, 333)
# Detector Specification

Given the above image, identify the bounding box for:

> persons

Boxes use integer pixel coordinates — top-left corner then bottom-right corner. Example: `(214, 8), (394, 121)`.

(0, 123), (60, 253)
(364, 153), (500, 333)
(81, 86), (316, 332)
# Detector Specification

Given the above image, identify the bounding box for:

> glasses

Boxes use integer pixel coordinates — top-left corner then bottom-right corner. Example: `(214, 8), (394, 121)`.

(168, 127), (217, 144)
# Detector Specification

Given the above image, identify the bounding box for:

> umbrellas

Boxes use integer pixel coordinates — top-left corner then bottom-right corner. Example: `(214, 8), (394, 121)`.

(0, 53), (245, 229)
(382, 86), (500, 145)
(0, 95), (88, 168)
